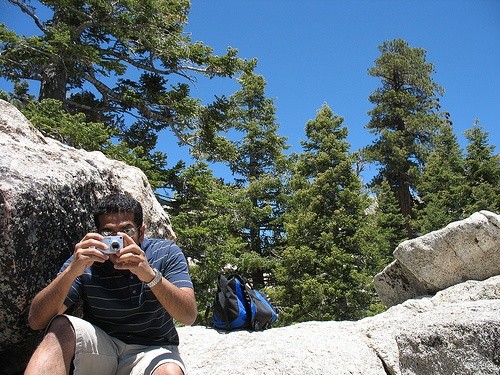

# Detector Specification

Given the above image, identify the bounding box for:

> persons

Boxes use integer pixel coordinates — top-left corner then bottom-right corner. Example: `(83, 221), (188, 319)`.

(23, 194), (198, 375)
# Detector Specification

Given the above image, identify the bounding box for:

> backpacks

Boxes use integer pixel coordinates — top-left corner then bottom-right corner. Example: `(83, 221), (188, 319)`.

(204, 272), (277, 335)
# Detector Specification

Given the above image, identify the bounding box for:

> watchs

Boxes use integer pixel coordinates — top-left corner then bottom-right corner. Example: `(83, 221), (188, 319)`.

(145, 269), (162, 288)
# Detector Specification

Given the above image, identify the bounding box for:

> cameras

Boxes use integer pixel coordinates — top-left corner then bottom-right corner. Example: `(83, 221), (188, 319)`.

(95, 236), (123, 254)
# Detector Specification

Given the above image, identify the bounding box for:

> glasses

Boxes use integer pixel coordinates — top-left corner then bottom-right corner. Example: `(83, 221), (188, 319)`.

(101, 226), (140, 238)
